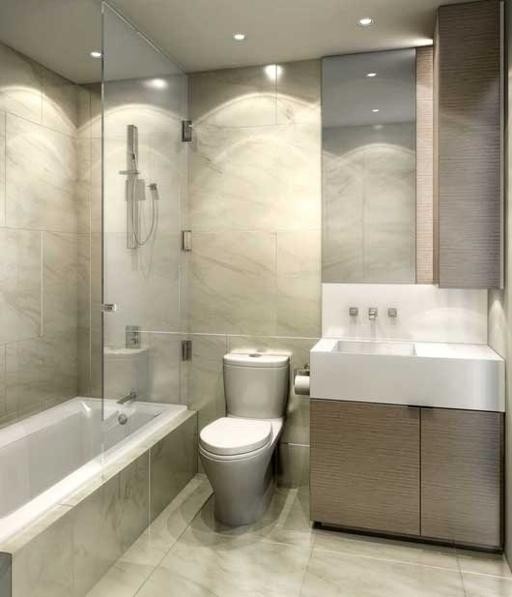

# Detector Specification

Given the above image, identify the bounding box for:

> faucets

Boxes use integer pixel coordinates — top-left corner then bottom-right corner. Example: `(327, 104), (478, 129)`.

(368, 307), (377, 321)
(115, 392), (136, 404)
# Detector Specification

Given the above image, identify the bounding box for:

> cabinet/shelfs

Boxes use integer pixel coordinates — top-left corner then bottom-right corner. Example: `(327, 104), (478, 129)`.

(304, 396), (506, 557)
(432, 3), (504, 289)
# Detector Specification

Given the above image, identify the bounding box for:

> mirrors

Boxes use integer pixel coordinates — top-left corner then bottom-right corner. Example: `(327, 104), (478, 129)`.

(320, 47), (419, 283)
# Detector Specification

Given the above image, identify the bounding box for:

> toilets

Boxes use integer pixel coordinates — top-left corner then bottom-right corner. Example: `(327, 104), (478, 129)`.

(196, 351), (293, 524)
(103, 345), (150, 404)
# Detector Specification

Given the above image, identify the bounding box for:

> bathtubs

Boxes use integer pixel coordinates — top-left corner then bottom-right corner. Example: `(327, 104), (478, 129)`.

(0, 395), (196, 597)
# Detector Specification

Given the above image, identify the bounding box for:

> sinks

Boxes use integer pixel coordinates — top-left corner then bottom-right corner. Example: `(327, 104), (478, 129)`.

(332, 340), (417, 357)
(415, 344), (505, 362)
